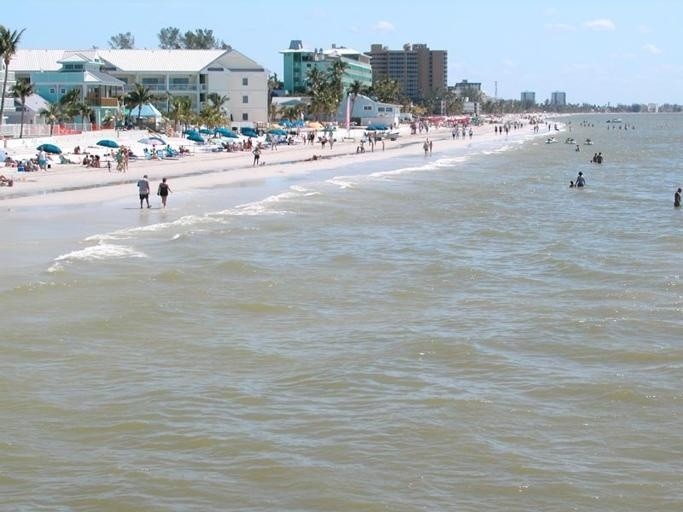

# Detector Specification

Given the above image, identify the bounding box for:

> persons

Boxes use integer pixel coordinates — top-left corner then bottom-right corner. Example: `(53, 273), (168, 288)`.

(607, 118), (627, 131)
(674, 187), (682, 207)
(529, 114), (595, 152)
(570, 171), (585, 188)
(0, 114), (189, 189)
(136, 174), (152, 209)
(590, 151), (605, 164)
(157, 177), (173, 208)
(207, 114), (523, 164)
